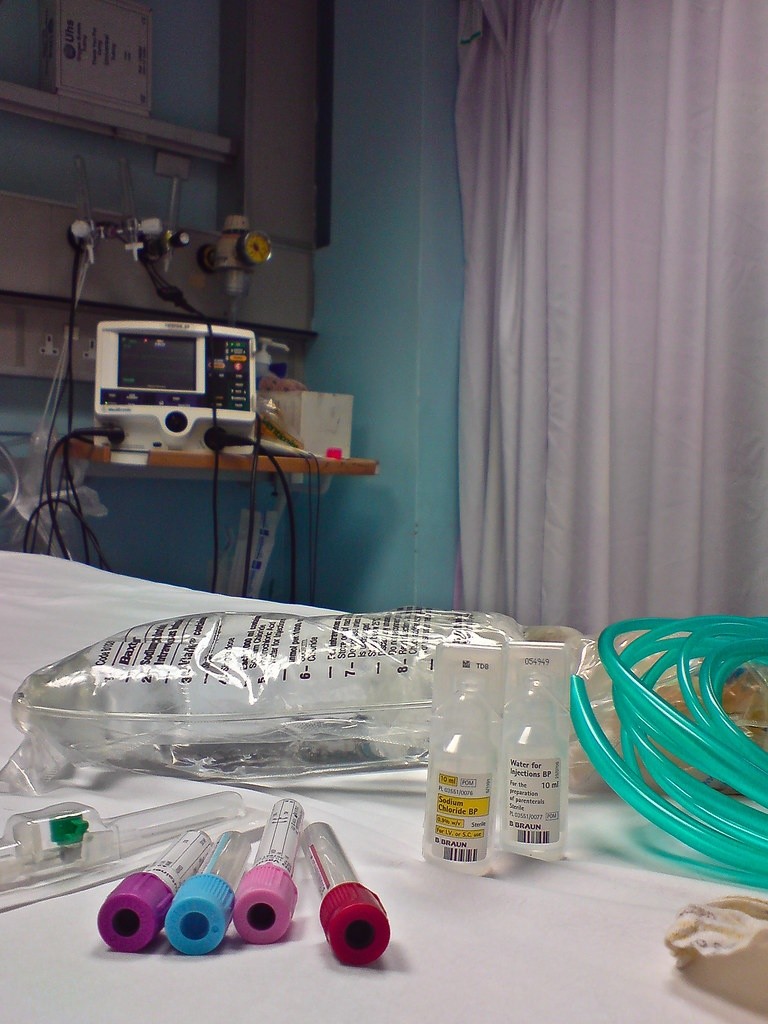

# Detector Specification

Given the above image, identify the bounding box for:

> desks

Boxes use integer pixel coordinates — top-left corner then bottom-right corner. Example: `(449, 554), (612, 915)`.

(58, 437), (383, 497)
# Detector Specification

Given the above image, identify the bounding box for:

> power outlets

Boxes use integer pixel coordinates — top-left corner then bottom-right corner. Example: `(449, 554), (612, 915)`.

(40, 325), (97, 369)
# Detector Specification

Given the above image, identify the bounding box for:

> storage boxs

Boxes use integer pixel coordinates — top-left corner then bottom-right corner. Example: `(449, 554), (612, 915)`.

(258, 391), (355, 457)
(38, 0), (152, 117)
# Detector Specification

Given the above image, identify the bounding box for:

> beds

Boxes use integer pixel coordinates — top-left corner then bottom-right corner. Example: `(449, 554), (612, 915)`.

(0, 547), (768, 1023)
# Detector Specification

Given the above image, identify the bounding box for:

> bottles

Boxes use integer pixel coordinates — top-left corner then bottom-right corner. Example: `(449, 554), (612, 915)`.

(422, 645), (573, 878)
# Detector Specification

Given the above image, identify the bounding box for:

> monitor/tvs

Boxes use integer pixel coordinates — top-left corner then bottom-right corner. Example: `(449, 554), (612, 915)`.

(116, 331), (197, 392)
